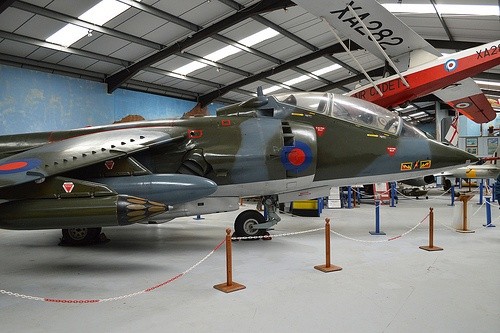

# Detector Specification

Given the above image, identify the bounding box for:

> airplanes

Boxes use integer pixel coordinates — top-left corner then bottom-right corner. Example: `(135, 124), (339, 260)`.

(0, 84), (499, 245)
(288, 0), (500, 125)
